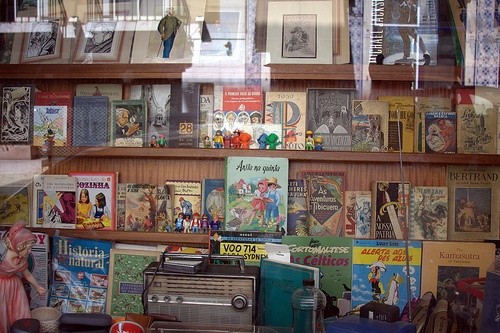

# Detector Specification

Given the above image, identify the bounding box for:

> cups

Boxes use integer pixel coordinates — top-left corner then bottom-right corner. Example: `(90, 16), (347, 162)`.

(31, 307), (62, 333)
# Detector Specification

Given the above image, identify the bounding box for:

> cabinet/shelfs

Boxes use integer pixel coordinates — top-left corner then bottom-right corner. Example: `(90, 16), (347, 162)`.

(0, 0), (500, 254)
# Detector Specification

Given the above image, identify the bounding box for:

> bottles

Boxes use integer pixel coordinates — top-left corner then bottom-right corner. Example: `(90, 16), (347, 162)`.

(291, 279), (327, 333)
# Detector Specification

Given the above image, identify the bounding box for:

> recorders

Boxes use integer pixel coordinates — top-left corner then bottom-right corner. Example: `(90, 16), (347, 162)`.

(144, 256), (260, 325)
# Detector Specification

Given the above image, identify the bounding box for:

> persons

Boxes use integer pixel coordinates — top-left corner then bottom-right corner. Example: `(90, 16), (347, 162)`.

(231, 130), (242, 148)
(201, 136), (211, 148)
(157, 133), (167, 147)
(223, 131), (232, 148)
(182, 214), (192, 233)
(190, 212), (201, 234)
(174, 213), (184, 233)
(199, 214), (210, 234)
(305, 131), (314, 150)
(398, 0), (432, 61)
(0, 218), (46, 333)
(149, 135), (157, 147)
(212, 130), (223, 149)
(210, 215), (221, 230)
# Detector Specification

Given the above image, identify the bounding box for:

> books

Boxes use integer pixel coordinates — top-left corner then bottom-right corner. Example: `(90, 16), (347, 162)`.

(0, 82), (500, 333)
(370, 0), (439, 65)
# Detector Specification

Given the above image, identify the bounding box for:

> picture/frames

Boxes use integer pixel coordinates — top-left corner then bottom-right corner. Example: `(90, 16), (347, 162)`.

(73, 10), (129, 62)
(0, 83), (35, 145)
(19, 15), (63, 64)
